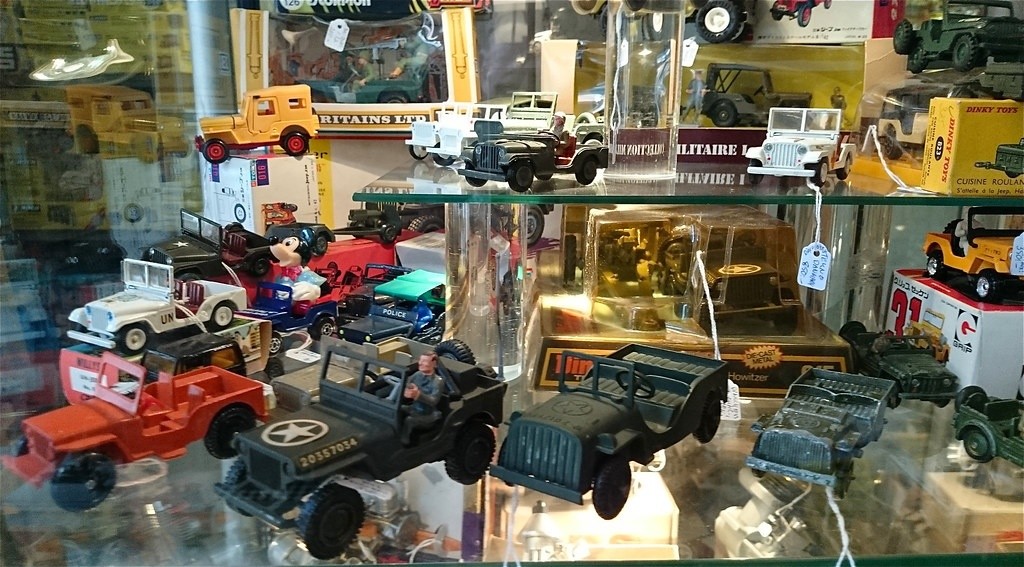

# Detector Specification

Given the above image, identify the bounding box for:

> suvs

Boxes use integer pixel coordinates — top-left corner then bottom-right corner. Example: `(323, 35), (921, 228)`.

(0, 348), (271, 515)
(214, 341), (508, 562)
(65, 258), (247, 357)
(839, 320), (956, 409)
(233, 280), (338, 355)
(365, 176), (556, 249)
(953, 385), (1024, 468)
(490, 340), (731, 523)
(335, 262), (415, 324)
(922, 206), (1024, 301)
(145, 208), (274, 284)
(338, 269), (448, 348)
(659, 231), (801, 336)
(458, 118), (610, 192)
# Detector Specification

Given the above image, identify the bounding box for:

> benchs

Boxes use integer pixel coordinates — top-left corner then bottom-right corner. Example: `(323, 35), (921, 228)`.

(985, 401), (1019, 432)
(429, 297), (445, 315)
(621, 351), (717, 385)
(292, 294), (333, 315)
(929, 19), (943, 42)
(814, 377), (886, 400)
(1011, 417), (1024, 445)
(857, 332), (883, 350)
(375, 383), (450, 447)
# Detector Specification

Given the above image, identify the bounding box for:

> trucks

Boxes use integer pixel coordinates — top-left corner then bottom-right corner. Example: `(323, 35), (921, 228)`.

(747, 368), (898, 499)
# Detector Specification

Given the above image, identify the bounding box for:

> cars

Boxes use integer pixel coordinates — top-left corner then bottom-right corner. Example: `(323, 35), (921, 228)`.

(357, 53), (446, 105)
(770, 0), (832, 28)
(66, 84), (191, 163)
(200, 82), (320, 162)
(703, 61), (812, 127)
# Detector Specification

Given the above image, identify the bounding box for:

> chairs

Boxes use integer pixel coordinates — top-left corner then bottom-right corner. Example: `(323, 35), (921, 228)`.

(395, 351), (412, 368)
(383, 274), (397, 281)
(624, 375), (689, 428)
(743, 95), (754, 104)
(554, 135), (577, 165)
(177, 282), (205, 318)
(579, 365), (636, 396)
(212, 226), (228, 241)
(788, 385), (835, 408)
(372, 47), (385, 78)
(832, 393), (880, 434)
(143, 371), (175, 429)
(391, 301), (434, 336)
(166, 277), (183, 301)
(222, 233), (246, 267)
(952, 227), (965, 257)
(160, 385), (205, 432)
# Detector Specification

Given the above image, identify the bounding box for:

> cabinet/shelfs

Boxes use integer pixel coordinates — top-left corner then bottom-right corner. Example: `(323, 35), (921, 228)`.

(1, 152), (1024, 566)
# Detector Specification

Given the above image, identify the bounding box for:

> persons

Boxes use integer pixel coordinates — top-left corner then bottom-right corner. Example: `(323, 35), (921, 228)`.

(548, 111), (570, 156)
(955, 205), (984, 256)
(332, 27), (429, 92)
(681, 70), (710, 125)
(401, 351), (444, 442)
(825, 85), (847, 130)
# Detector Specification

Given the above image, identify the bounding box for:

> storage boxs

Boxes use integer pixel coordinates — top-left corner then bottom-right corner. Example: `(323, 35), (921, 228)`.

(0, 0), (1024, 551)
